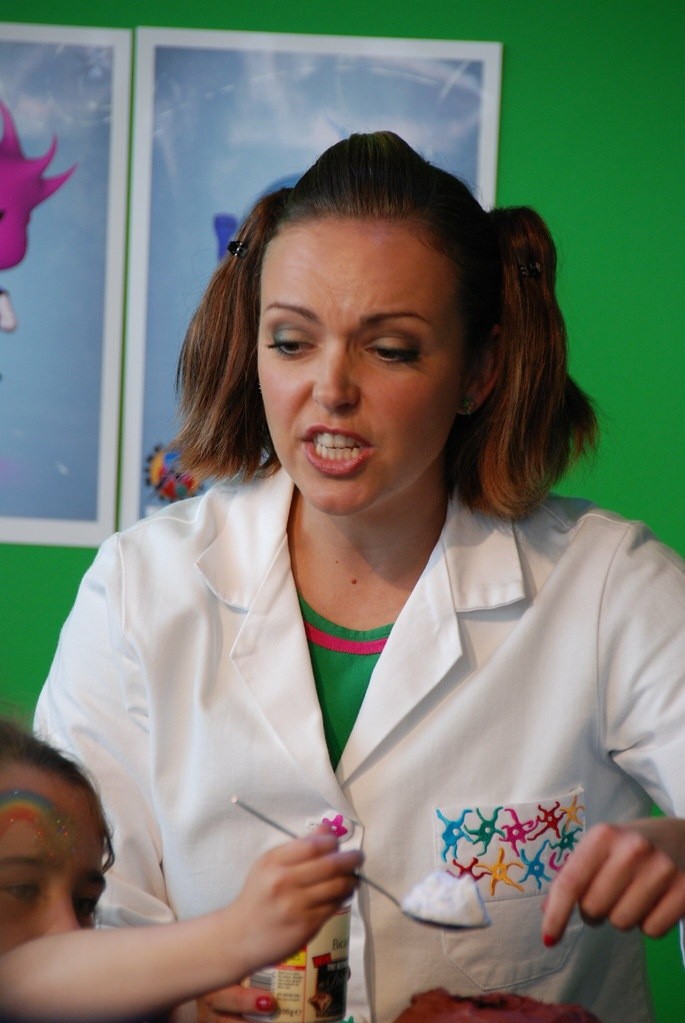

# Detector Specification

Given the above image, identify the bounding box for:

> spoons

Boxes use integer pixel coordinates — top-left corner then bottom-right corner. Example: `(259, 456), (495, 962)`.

(231, 796), (493, 931)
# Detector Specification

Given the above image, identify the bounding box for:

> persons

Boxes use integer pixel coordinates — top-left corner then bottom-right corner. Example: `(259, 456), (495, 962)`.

(34, 130), (684, 1023)
(0, 719), (368, 1023)
